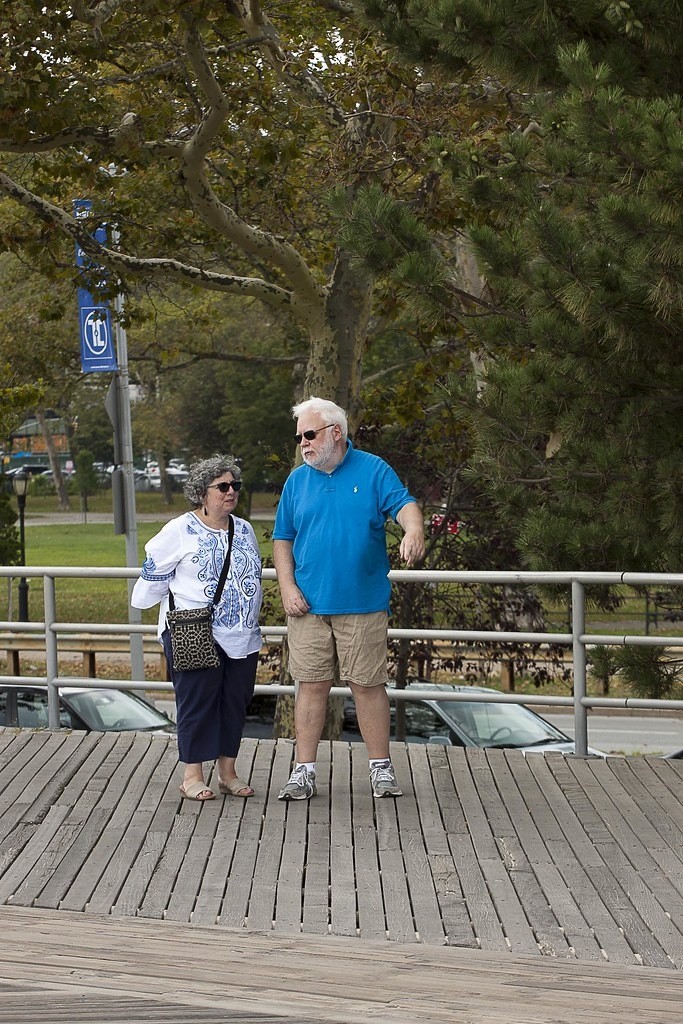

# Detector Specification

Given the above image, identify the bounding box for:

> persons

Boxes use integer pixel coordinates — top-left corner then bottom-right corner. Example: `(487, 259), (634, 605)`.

(131, 455), (262, 800)
(275, 398), (427, 800)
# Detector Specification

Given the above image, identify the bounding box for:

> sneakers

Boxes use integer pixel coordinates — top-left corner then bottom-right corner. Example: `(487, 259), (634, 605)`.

(369, 758), (403, 797)
(278, 766), (317, 800)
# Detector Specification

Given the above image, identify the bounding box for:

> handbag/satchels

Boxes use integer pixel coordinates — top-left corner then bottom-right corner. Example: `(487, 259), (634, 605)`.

(165, 608), (219, 671)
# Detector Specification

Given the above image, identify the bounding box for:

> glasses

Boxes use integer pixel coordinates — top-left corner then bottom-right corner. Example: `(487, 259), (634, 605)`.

(207, 480), (242, 493)
(292, 424), (335, 444)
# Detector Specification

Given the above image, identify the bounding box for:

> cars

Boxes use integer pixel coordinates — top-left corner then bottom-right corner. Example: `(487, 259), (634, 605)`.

(244, 682), (611, 757)
(5, 457), (190, 491)
(0, 684), (178, 735)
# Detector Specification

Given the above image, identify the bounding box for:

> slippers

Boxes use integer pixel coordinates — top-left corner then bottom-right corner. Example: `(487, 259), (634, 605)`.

(178, 782), (216, 800)
(218, 778), (255, 797)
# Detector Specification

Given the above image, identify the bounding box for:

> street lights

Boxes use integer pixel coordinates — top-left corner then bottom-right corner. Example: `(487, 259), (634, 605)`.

(11, 465), (33, 634)
